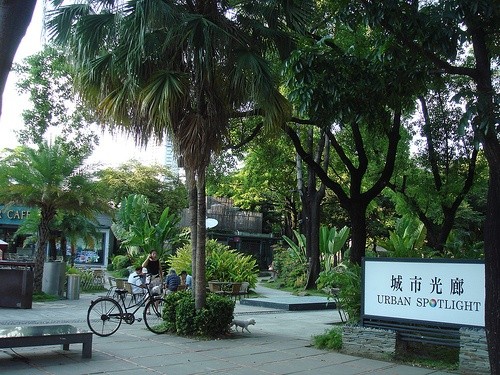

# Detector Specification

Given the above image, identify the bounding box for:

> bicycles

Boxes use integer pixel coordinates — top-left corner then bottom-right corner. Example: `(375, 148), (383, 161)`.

(87, 273), (174, 338)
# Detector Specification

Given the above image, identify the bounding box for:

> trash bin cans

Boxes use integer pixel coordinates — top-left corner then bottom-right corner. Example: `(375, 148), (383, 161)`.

(66, 274), (80, 300)
(0, 259), (35, 309)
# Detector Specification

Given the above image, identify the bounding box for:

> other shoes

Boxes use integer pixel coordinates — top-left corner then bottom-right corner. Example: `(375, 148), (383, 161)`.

(147, 312), (151, 315)
(156, 312), (161, 317)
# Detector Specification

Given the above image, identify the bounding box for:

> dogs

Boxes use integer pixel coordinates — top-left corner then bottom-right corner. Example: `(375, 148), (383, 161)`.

(230, 319), (256, 333)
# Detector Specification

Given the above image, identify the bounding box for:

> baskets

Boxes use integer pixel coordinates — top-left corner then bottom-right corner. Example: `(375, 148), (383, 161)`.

(149, 283), (163, 295)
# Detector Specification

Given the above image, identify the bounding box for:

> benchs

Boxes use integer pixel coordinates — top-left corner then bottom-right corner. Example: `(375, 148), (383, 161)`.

(0, 325), (94, 358)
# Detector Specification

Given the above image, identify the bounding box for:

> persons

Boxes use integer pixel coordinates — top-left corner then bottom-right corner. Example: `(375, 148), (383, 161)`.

(178, 271), (193, 290)
(141, 250), (162, 315)
(128, 266), (146, 307)
(163, 269), (180, 296)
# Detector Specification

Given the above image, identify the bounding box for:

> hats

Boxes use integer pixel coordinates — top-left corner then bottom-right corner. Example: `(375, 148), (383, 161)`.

(142, 268), (147, 273)
(178, 271), (186, 276)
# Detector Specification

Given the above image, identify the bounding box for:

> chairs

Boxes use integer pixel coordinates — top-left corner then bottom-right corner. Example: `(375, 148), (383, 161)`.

(106, 277), (250, 309)
